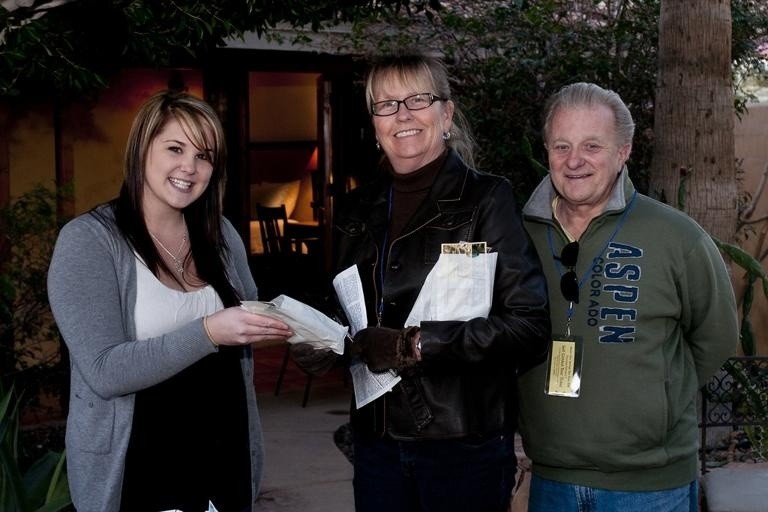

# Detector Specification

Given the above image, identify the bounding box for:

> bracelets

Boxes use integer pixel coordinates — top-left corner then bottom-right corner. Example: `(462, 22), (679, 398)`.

(415, 336), (421, 354)
(202, 315), (219, 347)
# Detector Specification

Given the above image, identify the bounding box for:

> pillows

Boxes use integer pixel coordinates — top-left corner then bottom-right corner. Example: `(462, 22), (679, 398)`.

(250, 178), (302, 217)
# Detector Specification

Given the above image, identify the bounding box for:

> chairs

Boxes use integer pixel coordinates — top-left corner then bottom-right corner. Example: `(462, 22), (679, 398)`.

(256, 203), (291, 278)
(273, 221), (330, 409)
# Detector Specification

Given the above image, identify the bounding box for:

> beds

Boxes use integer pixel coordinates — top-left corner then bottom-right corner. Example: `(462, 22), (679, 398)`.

(247, 174), (314, 253)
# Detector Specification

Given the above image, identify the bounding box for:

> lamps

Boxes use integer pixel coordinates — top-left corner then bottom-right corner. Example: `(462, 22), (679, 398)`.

(304, 144), (319, 220)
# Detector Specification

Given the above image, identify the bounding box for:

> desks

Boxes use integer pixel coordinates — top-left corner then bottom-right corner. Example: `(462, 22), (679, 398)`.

(280, 220), (322, 255)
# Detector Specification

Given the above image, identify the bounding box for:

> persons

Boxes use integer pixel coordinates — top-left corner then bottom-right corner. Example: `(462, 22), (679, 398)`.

(292, 47), (551, 512)
(508, 82), (739, 511)
(47, 92), (293, 512)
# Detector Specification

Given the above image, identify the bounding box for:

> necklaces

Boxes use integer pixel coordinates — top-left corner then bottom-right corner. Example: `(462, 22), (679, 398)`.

(150, 213), (186, 272)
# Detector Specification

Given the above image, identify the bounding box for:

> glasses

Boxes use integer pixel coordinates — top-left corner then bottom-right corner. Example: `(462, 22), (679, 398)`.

(552, 240), (580, 305)
(370, 92), (449, 118)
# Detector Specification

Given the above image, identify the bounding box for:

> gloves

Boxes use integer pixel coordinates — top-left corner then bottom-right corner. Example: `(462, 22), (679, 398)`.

(342, 325), (423, 375)
